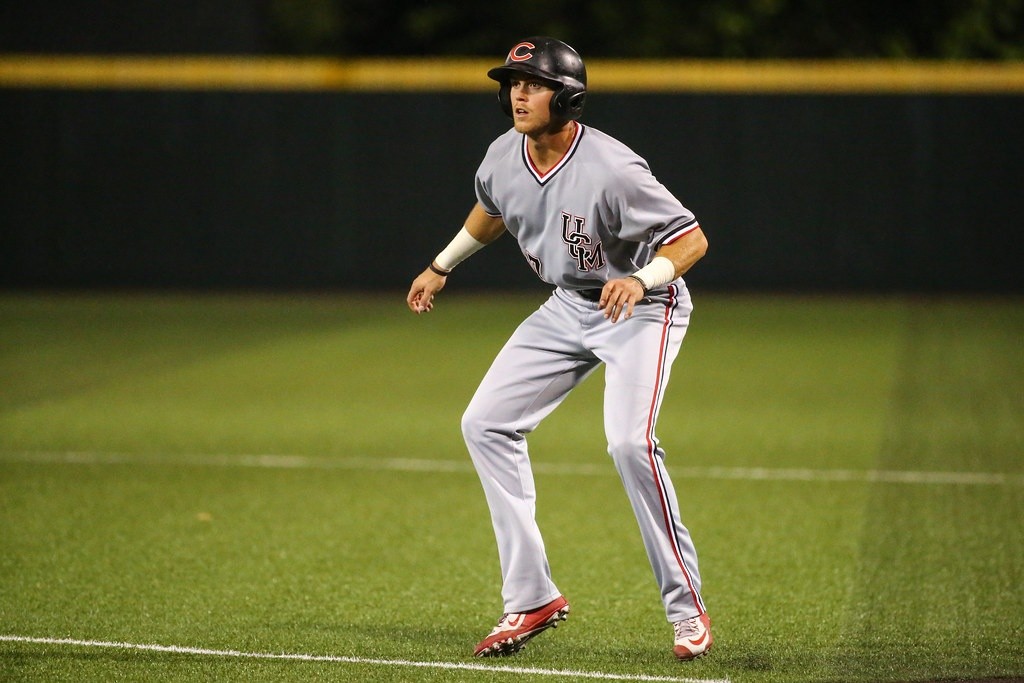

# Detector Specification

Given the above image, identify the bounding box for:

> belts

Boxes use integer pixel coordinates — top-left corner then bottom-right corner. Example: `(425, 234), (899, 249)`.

(575, 289), (602, 302)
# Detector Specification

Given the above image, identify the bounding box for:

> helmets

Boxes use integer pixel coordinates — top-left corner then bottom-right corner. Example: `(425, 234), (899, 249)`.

(487, 36), (587, 131)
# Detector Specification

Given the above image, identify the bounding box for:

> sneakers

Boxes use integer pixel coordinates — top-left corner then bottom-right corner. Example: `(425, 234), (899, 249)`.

(473, 595), (570, 659)
(673, 611), (713, 661)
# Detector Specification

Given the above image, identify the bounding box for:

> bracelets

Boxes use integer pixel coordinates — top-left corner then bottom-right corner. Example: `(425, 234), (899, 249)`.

(430, 260), (451, 276)
(629, 276), (651, 303)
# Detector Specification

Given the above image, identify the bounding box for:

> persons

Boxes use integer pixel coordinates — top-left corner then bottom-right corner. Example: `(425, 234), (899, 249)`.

(407, 36), (712, 660)
